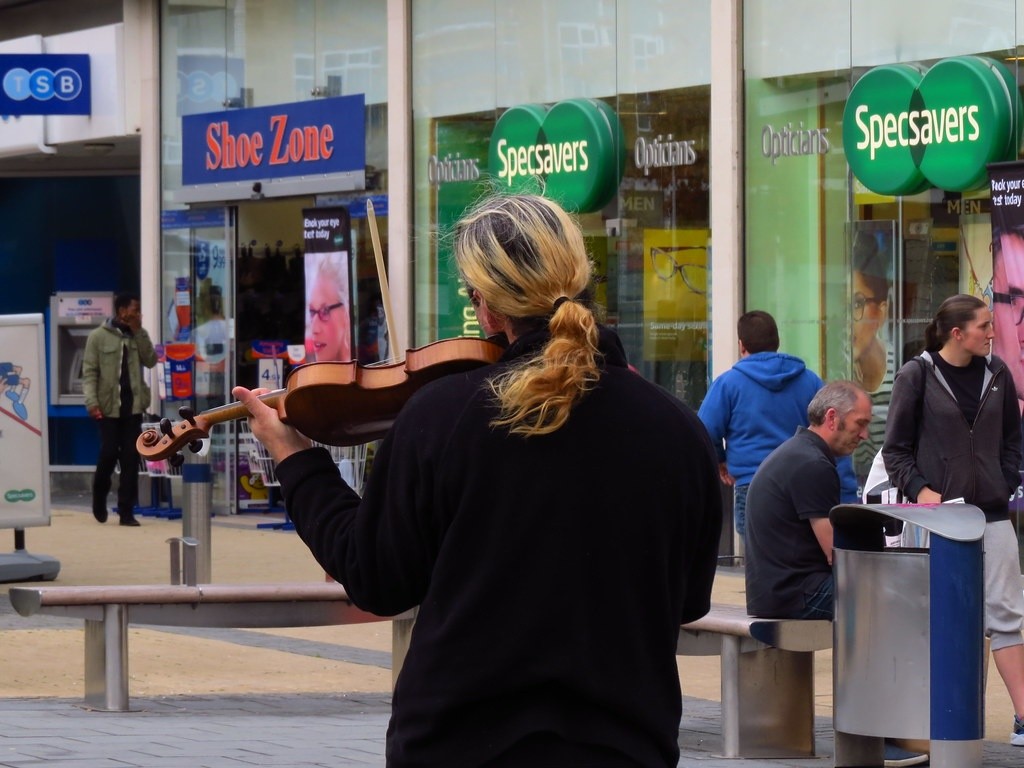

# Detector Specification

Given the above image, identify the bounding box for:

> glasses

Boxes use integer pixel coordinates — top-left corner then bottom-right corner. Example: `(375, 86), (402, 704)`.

(306, 303), (343, 322)
(851, 295), (879, 322)
(650, 244), (707, 295)
(993, 292), (1024, 325)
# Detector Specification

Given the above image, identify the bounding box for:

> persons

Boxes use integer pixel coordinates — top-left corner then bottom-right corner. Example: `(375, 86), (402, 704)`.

(853, 229), (895, 485)
(310, 259), (348, 365)
(883, 294), (1024, 745)
(745, 381), (929, 766)
(232, 194), (722, 768)
(697, 311), (857, 538)
(994, 224), (1024, 417)
(82, 292), (158, 526)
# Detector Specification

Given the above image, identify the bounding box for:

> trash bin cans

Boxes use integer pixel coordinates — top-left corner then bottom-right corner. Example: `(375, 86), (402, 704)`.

(831, 503), (988, 738)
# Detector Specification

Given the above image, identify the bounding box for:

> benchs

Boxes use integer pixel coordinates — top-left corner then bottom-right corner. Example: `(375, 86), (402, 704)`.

(8, 582), (420, 711)
(678, 600), (833, 759)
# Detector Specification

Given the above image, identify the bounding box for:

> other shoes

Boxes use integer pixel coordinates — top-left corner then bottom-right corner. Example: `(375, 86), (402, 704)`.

(93, 495), (108, 523)
(883, 741), (929, 767)
(120, 515), (140, 526)
(1010, 715), (1024, 746)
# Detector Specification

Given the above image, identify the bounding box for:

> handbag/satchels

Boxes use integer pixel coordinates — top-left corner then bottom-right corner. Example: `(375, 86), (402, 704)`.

(862, 445), (908, 537)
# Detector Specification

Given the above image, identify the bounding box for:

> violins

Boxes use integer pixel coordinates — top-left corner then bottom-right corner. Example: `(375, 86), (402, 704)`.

(134, 335), (506, 468)
